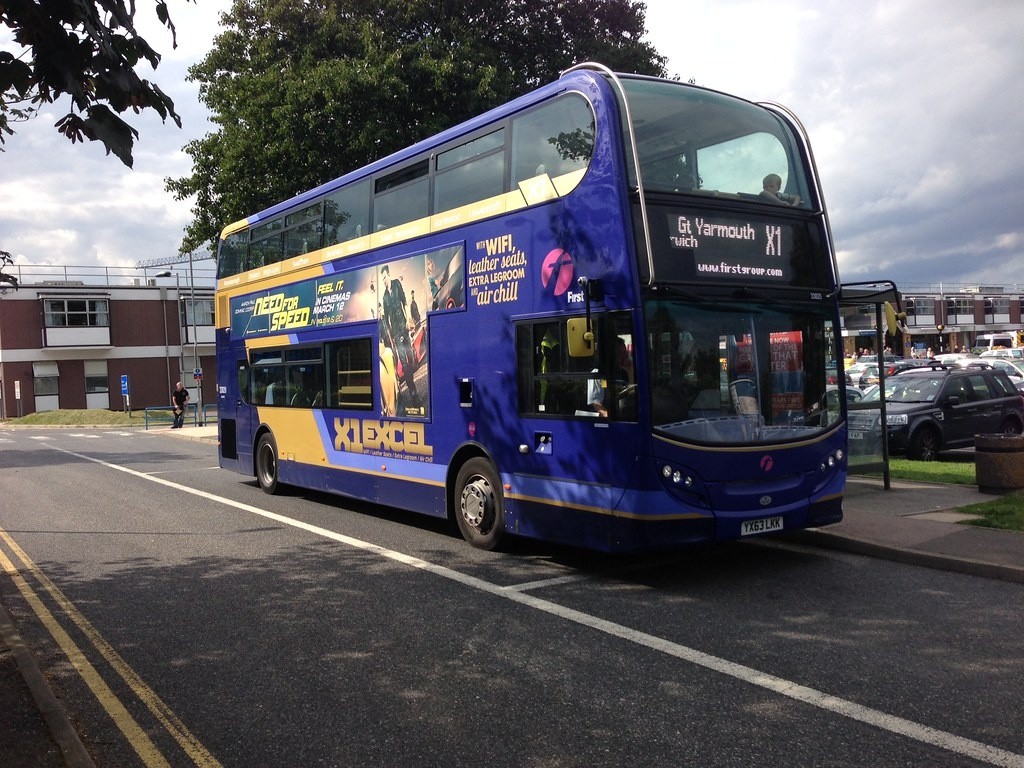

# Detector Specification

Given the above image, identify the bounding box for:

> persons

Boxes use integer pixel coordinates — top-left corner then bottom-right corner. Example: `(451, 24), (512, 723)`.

(264, 378), (293, 405)
(844, 346), (892, 360)
(960, 346), (968, 352)
(910, 346), (935, 360)
(171, 382), (190, 429)
(954, 346), (960, 353)
(586, 338), (634, 417)
(677, 173), (696, 192)
(761, 174), (800, 206)
(379, 264), (423, 407)
(945, 343), (951, 351)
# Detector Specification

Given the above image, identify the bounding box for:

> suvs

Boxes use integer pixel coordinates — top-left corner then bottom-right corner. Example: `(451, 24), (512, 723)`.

(843, 364), (1024, 461)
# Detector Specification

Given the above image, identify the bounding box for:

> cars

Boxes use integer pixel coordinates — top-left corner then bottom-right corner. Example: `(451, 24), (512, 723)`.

(824, 348), (1024, 433)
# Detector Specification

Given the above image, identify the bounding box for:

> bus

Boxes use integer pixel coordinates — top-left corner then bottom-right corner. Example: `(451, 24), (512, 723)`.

(214, 60), (849, 549)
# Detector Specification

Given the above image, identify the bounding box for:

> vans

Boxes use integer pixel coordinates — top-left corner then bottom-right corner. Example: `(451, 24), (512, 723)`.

(972, 334), (1012, 351)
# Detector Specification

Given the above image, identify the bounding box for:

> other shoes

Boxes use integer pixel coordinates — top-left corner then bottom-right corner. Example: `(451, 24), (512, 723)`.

(171, 426), (178, 428)
(179, 425), (182, 427)
